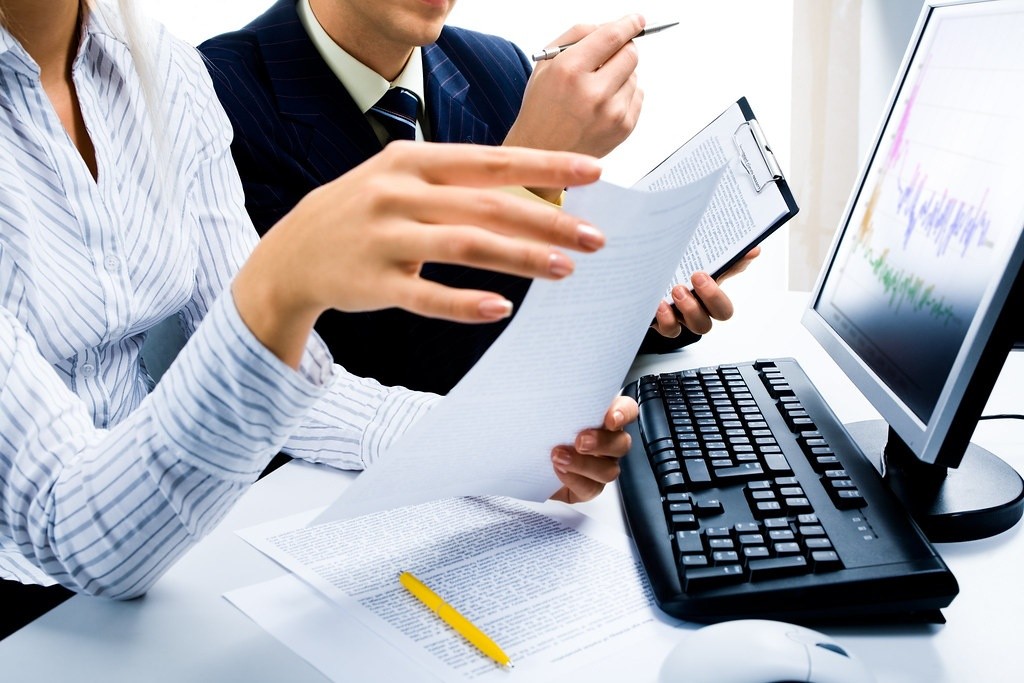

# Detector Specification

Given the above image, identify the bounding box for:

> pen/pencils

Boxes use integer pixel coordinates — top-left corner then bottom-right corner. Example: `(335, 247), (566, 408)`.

(397, 570), (515, 671)
(531, 20), (681, 62)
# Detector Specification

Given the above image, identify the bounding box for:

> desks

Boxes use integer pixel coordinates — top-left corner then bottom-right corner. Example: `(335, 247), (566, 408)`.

(2, 291), (1024, 683)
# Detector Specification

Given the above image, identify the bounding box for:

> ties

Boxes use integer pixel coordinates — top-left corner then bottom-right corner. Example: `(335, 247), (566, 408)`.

(368, 88), (421, 140)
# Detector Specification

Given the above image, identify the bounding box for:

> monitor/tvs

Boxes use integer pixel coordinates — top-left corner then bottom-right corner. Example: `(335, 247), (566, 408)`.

(799, 0), (1024, 543)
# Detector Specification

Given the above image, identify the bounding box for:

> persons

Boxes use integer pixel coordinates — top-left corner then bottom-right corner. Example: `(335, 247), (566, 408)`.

(0, 0), (642, 638)
(196, 0), (761, 402)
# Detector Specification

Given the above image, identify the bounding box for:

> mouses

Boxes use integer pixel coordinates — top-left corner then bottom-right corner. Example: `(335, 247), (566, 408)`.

(654, 619), (868, 683)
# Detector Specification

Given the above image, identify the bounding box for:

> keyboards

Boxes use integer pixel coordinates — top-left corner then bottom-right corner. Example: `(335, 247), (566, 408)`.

(616, 358), (960, 626)
